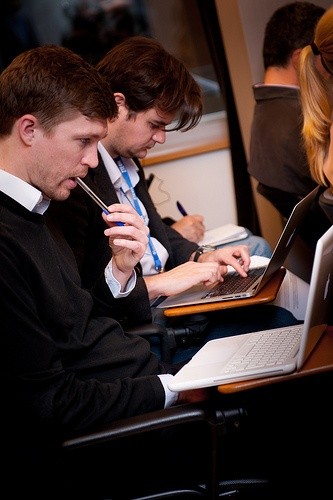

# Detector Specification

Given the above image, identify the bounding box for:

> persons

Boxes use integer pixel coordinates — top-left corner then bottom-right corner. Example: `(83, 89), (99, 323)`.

(58, 36), (297, 375)
(0, 48), (325, 500)
(248, 1), (333, 204)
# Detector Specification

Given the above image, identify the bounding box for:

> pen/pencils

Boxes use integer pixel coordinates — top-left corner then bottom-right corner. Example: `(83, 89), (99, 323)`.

(177, 201), (187, 216)
(75, 177), (124, 226)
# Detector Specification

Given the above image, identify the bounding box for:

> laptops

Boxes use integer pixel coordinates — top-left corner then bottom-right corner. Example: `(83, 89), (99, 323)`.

(179, 225), (333, 391)
(156, 184), (321, 310)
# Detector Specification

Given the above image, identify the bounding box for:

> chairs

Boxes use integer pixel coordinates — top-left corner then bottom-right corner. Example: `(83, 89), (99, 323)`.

(0, 266), (332, 499)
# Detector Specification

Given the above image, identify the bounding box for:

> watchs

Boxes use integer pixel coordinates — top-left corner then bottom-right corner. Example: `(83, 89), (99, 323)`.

(193, 245), (215, 262)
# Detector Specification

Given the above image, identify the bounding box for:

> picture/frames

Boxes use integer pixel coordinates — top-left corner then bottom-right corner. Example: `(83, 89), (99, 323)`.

(3, 0), (242, 166)
